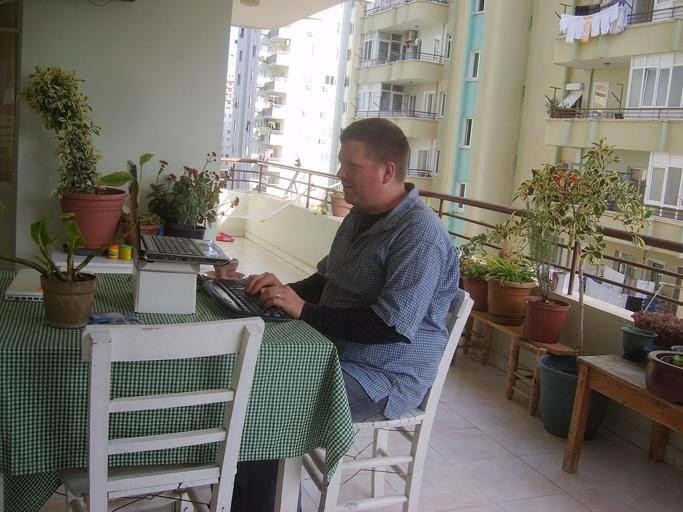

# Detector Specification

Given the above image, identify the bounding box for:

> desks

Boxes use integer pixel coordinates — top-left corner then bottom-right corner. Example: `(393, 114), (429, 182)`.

(563, 352), (683, 475)
(0, 266), (354, 512)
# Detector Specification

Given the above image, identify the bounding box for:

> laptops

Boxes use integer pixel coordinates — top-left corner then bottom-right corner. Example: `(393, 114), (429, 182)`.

(128, 158), (232, 266)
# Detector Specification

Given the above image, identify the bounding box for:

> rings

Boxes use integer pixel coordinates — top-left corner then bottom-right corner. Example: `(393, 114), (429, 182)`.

(277, 292), (280, 299)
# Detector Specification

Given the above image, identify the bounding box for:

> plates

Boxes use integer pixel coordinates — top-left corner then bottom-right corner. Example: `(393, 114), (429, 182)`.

(205, 271), (245, 282)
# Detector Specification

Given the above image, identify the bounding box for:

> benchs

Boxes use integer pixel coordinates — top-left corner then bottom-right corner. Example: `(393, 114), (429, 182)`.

(457, 312), (576, 417)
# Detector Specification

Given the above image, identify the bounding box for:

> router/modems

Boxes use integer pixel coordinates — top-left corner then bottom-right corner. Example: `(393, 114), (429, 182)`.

(5, 268), (45, 301)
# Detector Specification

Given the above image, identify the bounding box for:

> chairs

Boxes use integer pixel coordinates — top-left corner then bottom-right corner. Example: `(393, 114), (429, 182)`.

(56, 317), (266, 512)
(297, 288), (475, 512)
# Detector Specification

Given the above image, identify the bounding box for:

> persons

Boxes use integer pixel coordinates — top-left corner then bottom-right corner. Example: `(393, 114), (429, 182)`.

(232, 118), (459, 512)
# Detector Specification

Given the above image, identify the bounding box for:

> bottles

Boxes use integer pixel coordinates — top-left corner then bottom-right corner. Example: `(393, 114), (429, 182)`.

(110, 244), (132, 260)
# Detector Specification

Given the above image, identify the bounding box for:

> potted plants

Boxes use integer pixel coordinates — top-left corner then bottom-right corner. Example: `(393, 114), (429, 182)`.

(485, 209), (539, 327)
(121, 211), (161, 247)
(512, 134), (651, 441)
(320, 188), (353, 219)
(0, 154), (155, 330)
(456, 232), (491, 311)
(644, 351), (682, 406)
(519, 198), (571, 345)
(16, 66), (128, 249)
(146, 160), (176, 236)
(622, 310), (659, 362)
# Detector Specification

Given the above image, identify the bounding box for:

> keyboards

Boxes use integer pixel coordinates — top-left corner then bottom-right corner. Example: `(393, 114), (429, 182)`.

(202, 277), (288, 322)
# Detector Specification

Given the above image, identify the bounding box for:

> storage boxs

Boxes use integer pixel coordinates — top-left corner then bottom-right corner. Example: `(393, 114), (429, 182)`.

(131, 247), (200, 314)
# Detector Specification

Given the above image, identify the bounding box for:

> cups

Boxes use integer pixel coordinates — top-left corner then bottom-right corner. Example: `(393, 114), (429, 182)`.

(214, 257), (239, 274)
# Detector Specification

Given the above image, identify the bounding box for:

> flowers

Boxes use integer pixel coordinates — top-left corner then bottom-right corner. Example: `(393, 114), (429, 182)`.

(170, 151), (239, 228)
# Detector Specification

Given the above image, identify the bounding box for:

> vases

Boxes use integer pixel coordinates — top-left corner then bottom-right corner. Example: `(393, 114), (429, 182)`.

(171, 225), (207, 240)
